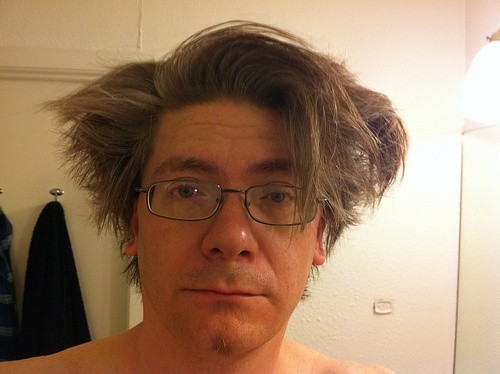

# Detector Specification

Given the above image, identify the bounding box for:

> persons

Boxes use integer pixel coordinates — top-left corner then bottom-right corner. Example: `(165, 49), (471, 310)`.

(0, 20), (408, 374)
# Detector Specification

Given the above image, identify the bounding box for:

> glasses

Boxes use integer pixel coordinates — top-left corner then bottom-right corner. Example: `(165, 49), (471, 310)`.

(136, 177), (332, 226)
